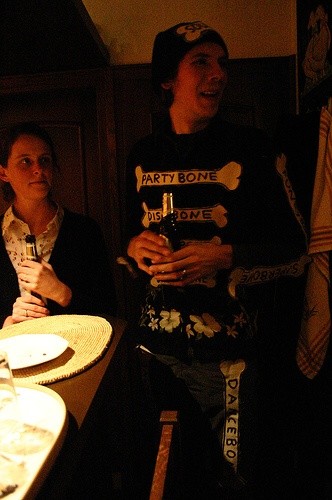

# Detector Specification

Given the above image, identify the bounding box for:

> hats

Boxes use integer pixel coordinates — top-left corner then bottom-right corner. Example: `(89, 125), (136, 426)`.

(150, 20), (230, 82)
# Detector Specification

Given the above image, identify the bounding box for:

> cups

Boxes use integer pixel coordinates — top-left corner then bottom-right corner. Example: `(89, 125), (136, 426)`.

(0, 350), (24, 433)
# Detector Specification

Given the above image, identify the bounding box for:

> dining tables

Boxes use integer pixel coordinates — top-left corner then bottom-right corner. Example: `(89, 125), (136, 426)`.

(43, 317), (129, 500)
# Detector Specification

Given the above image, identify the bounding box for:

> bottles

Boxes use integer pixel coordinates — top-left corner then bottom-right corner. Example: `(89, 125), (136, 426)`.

(25, 234), (42, 299)
(152, 192), (188, 298)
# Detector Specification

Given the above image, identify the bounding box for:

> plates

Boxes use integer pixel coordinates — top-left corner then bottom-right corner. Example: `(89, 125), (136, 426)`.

(0, 334), (68, 368)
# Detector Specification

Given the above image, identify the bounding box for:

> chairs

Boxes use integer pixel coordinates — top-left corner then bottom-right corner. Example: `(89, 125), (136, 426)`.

(149, 411), (184, 500)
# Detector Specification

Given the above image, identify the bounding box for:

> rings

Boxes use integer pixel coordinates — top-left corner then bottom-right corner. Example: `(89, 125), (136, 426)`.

(183, 270), (186, 274)
(25, 310), (28, 317)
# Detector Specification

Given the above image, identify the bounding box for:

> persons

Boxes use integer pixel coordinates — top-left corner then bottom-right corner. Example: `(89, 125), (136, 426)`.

(121, 20), (301, 500)
(0, 122), (104, 328)
